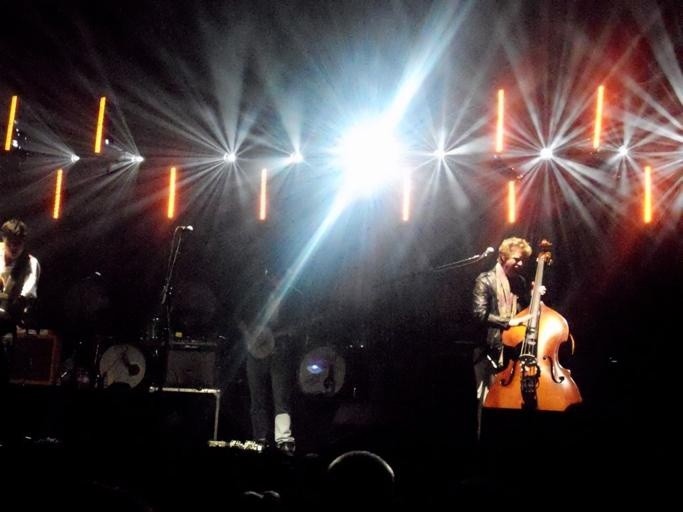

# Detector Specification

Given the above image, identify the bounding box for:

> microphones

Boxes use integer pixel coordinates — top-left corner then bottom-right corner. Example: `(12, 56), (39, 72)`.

(478, 246), (494, 261)
(177, 225), (194, 231)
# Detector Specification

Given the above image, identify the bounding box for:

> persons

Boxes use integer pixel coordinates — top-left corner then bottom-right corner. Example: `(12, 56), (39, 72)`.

(463, 236), (546, 388)
(0, 219), (41, 328)
(233, 268), (302, 452)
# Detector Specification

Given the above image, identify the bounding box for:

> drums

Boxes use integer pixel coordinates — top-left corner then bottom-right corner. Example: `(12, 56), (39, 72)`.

(99, 342), (147, 389)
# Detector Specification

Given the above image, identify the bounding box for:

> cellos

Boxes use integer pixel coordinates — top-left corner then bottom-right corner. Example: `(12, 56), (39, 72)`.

(482, 239), (581, 411)
